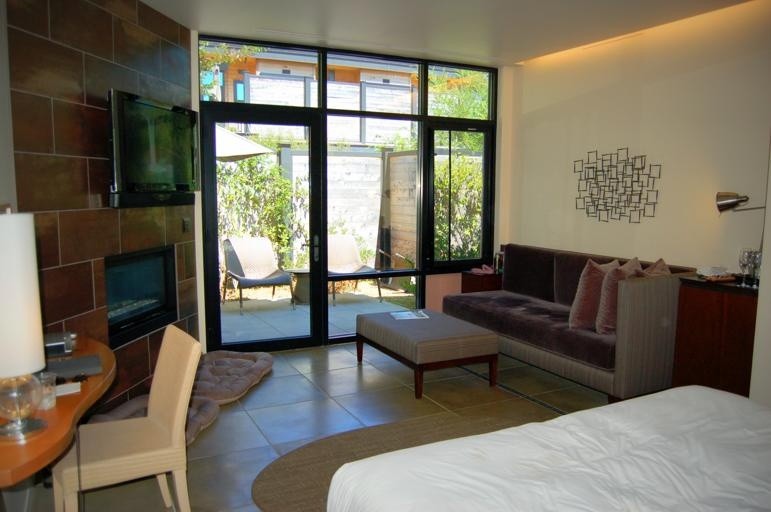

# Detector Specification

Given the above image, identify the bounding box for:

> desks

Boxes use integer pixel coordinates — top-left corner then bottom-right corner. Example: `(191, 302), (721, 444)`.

(0, 327), (118, 491)
(286, 267), (316, 306)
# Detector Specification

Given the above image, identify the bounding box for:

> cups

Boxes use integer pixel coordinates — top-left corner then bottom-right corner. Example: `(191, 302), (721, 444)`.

(31, 373), (57, 411)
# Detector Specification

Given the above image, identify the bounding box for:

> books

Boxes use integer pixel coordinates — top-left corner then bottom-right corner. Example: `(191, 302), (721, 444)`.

(31, 354), (102, 385)
(390, 310), (429, 320)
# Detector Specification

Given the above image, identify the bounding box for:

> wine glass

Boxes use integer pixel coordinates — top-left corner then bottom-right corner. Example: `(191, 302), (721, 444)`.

(737, 248), (761, 289)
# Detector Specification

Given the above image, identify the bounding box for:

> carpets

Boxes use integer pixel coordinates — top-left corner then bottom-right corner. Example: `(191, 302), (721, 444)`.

(250, 408), (537, 511)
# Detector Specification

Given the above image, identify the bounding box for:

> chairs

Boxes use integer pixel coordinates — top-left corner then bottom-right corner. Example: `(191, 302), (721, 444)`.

(45, 320), (204, 511)
(222, 236), (299, 316)
(327, 232), (386, 306)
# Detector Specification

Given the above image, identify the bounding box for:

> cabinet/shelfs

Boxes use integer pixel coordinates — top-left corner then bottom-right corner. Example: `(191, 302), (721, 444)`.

(461, 265), (502, 295)
(674, 274), (761, 399)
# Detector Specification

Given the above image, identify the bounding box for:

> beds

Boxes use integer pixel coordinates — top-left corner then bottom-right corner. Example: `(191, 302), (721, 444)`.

(325, 381), (771, 511)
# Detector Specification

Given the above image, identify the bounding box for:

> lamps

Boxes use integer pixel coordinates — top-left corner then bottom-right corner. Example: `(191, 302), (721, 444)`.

(0, 208), (52, 442)
(714, 192), (767, 255)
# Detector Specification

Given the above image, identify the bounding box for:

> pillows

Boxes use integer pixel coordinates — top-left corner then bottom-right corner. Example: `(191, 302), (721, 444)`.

(628, 256), (675, 278)
(595, 255), (643, 334)
(568, 257), (623, 331)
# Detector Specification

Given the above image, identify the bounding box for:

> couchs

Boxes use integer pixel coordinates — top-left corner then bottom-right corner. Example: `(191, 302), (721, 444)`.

(442, 241), (698, 405)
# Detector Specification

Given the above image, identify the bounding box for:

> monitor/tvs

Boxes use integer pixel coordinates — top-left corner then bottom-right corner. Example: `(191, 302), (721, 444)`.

(108, 88), (201, 208)
